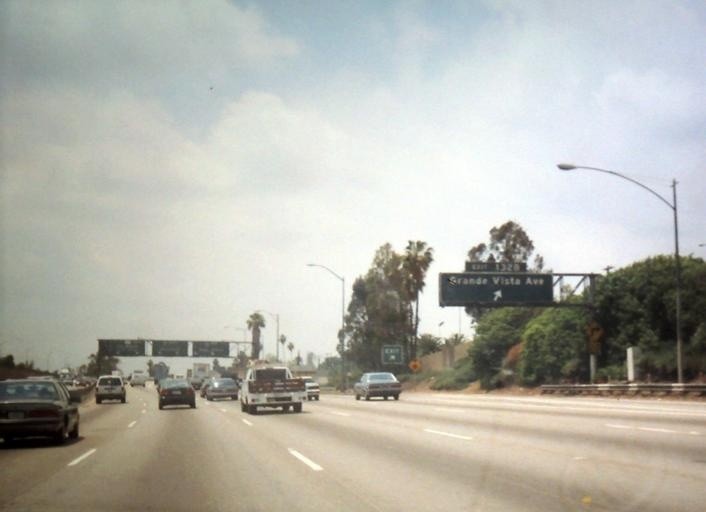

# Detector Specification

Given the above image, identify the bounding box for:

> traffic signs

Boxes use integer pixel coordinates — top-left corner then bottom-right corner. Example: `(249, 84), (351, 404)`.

(439, 273), (554, 305)
(381, 345), (403, 365)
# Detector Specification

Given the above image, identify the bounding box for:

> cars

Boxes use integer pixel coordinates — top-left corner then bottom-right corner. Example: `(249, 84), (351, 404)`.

(1, 374), (125, 444)
(354, 373), (401, 400)
(130, 370), (145, 387)
(297, 376), (320, 400)
(157, 371), (239, 409)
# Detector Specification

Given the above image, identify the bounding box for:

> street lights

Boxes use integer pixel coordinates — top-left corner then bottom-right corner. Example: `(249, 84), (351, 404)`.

(308, 263), (345, 394)
(558, 162), (684, 383)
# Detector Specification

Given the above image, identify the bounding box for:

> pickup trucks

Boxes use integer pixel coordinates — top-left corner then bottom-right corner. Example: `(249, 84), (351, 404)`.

(238, 367), (305, 414)
